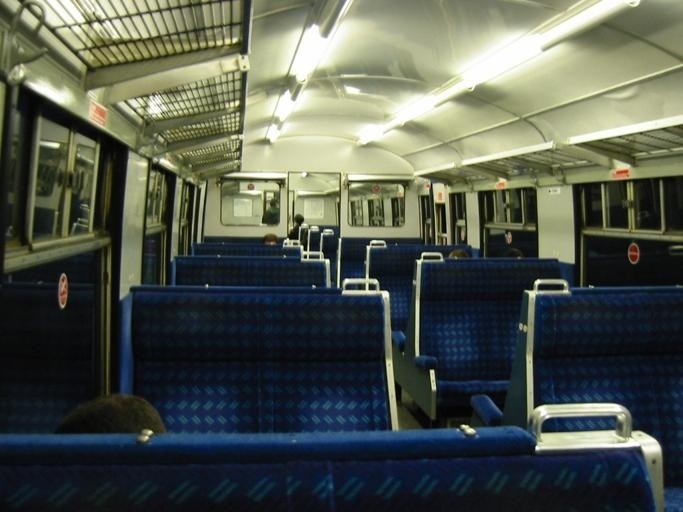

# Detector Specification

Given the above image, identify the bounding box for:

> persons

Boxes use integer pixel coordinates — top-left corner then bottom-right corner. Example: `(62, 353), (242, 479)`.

(502, 248), (525, 259)
(262, 200), (279, 221)
(286, 212), (304, 239)
(263, 233), (279, 245)
(52, 392), (165, 434)
(448, 248), (468, 257)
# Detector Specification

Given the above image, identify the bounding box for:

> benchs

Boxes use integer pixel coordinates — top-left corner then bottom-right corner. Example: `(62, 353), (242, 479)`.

(0, 222), (681, 509)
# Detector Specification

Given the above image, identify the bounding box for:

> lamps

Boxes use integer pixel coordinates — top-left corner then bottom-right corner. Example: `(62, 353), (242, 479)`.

(255, 0), (356, 148)
(354, 0), (643, 147)
